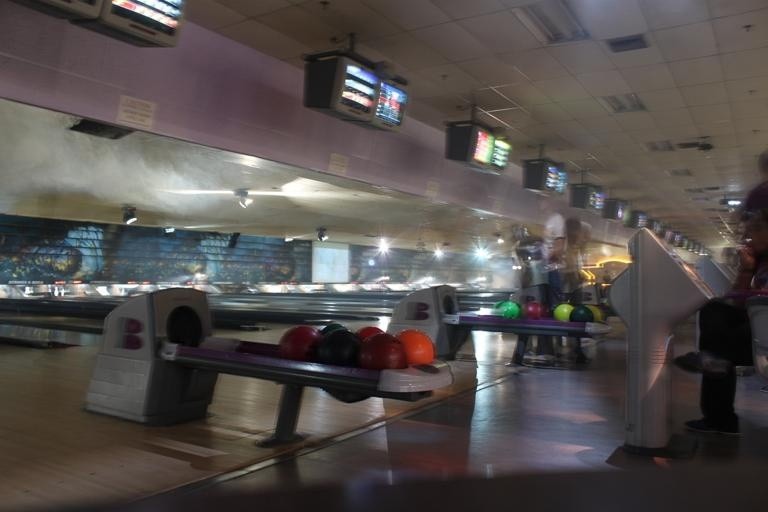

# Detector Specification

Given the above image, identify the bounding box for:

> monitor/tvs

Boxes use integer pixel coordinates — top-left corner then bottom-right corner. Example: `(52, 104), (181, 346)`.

(444, 124), (512, 176)
(17, 0), (185, 46)
(300, 53), (410, 132)
(570, 183), (713, 258)
(522, 158), (568, 198)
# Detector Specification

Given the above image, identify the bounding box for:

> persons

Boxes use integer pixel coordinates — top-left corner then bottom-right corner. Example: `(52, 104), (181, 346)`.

(671, 151), (768, 438)
(506, 196), (593, 366)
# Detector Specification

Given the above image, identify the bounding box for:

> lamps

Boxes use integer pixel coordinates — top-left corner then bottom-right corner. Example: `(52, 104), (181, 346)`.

(162, 226), (178, 237)
(121, 205), (139, 226)
(234, 190), (253, 208)
(316, 228), (328, 242)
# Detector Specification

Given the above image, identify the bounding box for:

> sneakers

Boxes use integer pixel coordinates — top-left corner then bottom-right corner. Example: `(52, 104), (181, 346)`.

(684, 416), (741, 434)
(675, 352), (754, 377)
